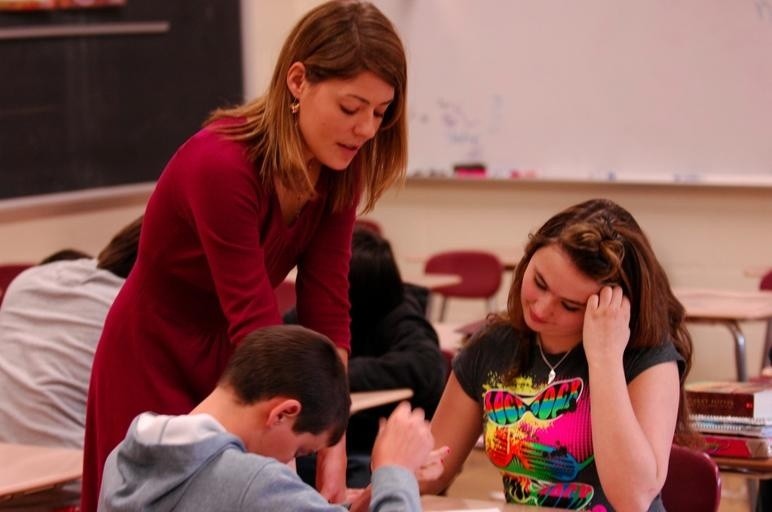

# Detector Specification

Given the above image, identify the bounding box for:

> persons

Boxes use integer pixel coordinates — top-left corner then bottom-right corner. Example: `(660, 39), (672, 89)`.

(415, 197), (707, 512)
(75, 0), (407, 510)
(40, 250), (92, 260)
(0, 213), (144, 510)
(96, 326), (436, 512)
(280, 226), (446, 487)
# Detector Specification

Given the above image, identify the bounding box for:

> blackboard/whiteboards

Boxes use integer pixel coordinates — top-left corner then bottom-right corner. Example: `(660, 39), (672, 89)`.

(2, 1), (244, 218)
(244, 0), (772, 192)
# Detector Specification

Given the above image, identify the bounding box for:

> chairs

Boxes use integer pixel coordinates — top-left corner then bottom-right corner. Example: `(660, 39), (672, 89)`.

(423, 249), (502, 327)
(658, 441), (721, 512)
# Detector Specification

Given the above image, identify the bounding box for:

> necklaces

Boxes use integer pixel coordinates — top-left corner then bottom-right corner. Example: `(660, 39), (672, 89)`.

(536, 331), (578, 384)
(288, 159), (313, 221)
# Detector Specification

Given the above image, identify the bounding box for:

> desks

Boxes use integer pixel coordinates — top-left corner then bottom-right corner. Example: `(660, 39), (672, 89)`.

(2, 439), (86, 511)
(672, 287), (771, 383)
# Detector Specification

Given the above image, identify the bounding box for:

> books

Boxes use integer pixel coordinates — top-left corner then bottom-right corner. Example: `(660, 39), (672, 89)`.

(686, 376), (772, 415)
(687, 414), (772, 459)
